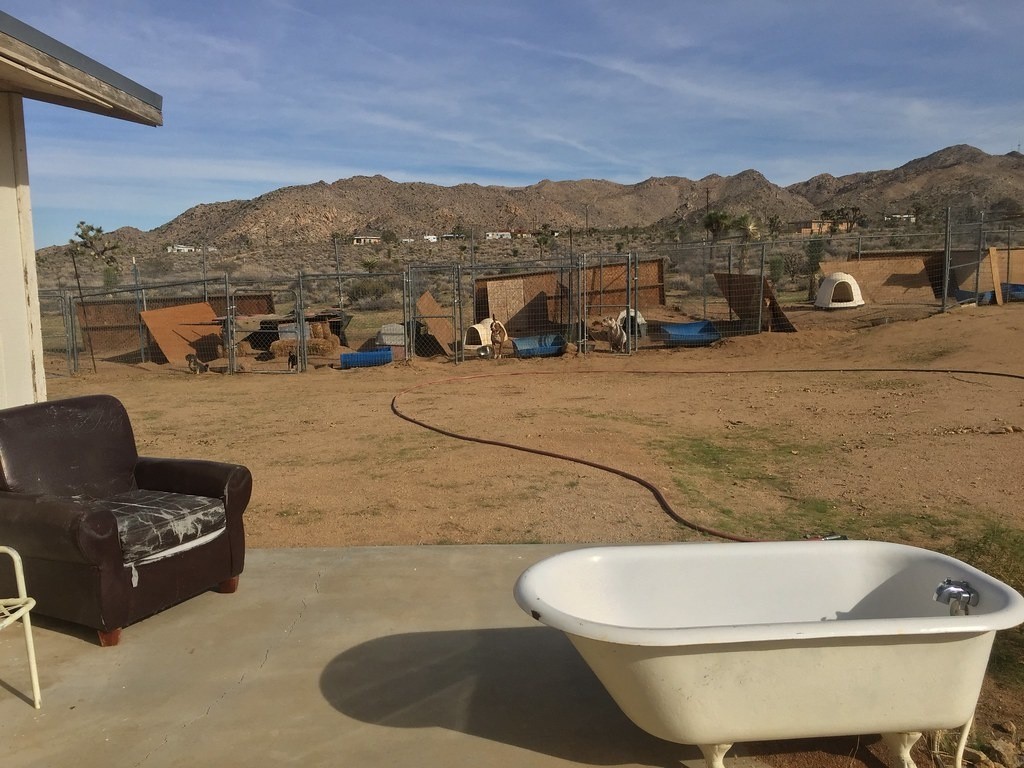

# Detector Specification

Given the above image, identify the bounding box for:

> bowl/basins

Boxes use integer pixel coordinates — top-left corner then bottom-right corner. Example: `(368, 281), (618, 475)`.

(477, 346), (493, 359)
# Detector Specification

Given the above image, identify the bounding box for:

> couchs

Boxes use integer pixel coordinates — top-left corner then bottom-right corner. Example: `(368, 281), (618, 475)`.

(0, 395), (255, 647)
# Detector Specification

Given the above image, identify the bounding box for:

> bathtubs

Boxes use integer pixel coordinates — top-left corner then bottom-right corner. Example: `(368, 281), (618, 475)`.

(514, 540), (1024, 768)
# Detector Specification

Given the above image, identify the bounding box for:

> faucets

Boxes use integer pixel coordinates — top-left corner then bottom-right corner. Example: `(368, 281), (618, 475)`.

(931, 587), (971, 606)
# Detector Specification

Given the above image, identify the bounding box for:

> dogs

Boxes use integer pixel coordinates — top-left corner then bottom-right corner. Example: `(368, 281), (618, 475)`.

(600, 316), (627, 353)
(185, 353), (210, 374)
(490, 314), (506, 358)
(287, 351), (299, 371)
